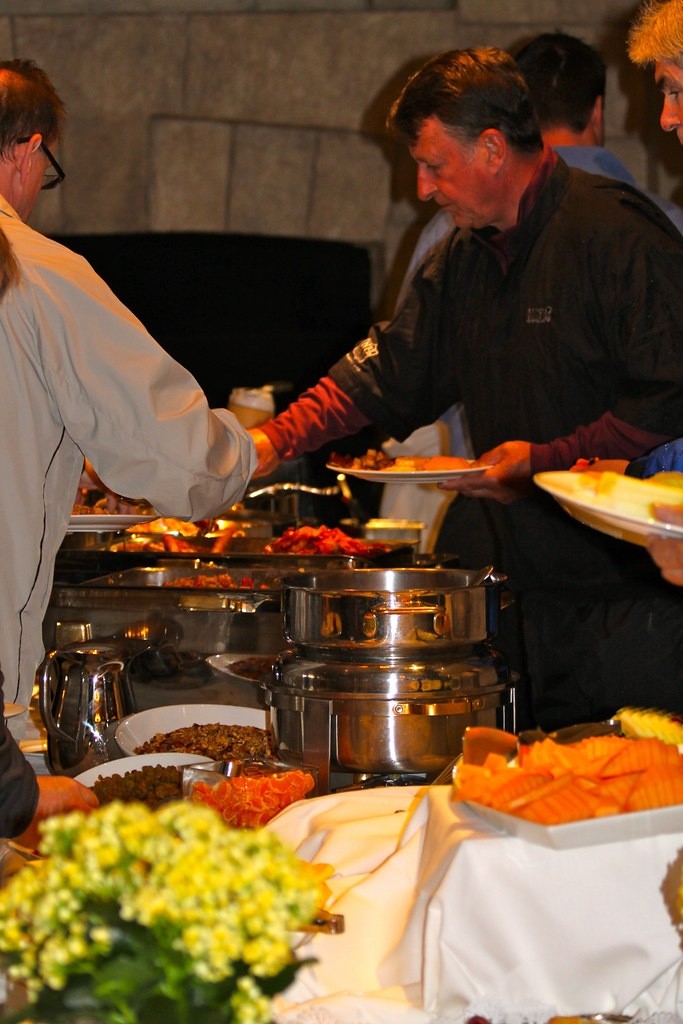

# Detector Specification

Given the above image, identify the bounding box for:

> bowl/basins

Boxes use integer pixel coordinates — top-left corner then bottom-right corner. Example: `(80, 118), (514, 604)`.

(70, 754), (215, 806)
(115, 704), (269, 757)
(364, 519), (423, 554)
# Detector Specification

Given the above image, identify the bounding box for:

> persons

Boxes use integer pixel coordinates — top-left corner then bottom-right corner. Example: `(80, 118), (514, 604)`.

(0, 693), (99, 850)
(381, 31), (683, 555)
(250, 47), (683, 730)
(571, 0), (683, 587)
(0, 59), (261, 710)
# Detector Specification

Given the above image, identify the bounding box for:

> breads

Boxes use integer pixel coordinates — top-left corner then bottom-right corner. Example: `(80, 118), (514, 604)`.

(571, 471), (683, 518)
(383, 456), (472, 470)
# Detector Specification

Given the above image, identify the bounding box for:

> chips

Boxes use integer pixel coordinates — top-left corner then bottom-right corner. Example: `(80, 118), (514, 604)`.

(451, 710), (683, 825)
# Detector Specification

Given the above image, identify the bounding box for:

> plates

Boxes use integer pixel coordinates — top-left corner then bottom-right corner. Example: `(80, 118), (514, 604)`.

(66, 516), (156, 532)
(205, 654), (275, 682)
(327, 459), (493, 483)
(532, 470), (683, 545)
(450, 756), (683, 848)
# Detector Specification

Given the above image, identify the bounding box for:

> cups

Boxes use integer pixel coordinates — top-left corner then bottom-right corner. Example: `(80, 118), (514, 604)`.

(229, 389), (274, 430)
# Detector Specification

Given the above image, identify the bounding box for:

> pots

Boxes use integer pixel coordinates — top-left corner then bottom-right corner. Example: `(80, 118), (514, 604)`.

(262, 567), (517, 775)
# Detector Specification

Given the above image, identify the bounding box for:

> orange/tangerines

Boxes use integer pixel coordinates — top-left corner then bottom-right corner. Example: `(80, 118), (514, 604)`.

(191, 770), (316, 830)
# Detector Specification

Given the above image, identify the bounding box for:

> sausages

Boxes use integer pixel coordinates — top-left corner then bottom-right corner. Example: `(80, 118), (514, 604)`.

(95, 494), (138, 514)
(144, 532), (235, 553)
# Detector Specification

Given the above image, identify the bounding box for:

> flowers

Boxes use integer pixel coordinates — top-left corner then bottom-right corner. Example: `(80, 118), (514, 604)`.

(0, 799), (340, 1024)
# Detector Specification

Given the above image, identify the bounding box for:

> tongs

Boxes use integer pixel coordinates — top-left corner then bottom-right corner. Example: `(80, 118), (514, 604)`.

(518, 720), (622, 745)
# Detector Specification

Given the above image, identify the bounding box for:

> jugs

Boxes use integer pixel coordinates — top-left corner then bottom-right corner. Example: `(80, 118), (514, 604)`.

(40, 637), (156, 776)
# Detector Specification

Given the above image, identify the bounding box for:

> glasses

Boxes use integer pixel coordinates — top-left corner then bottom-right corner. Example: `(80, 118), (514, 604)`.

(16, 135), (65, 190)
